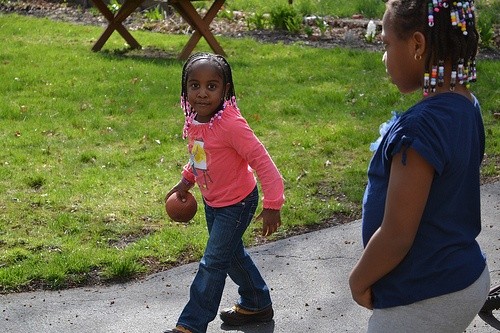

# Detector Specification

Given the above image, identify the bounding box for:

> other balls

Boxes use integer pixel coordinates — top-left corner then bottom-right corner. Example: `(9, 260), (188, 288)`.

(164, 189), (198, 223)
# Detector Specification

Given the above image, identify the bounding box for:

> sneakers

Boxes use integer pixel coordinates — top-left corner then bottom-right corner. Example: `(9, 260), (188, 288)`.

(220, 303), (274, 326)
(164, 324), (196, 333)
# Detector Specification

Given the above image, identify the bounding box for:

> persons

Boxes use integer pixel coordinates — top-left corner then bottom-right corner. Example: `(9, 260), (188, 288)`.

(346, 0), (490, 333)
(161, 52), (284, 333)
